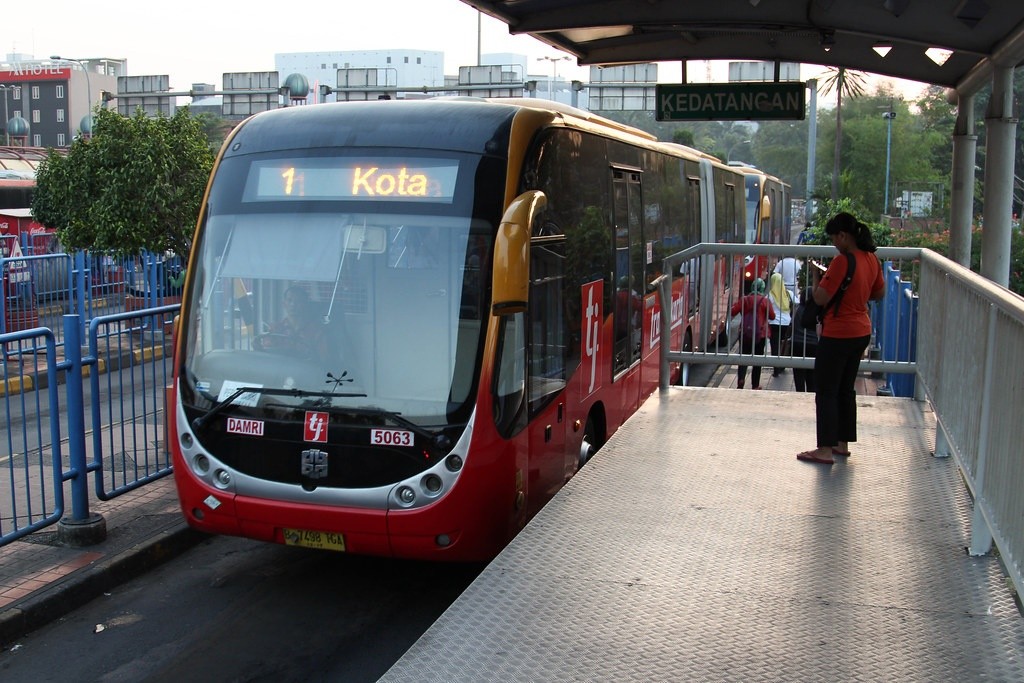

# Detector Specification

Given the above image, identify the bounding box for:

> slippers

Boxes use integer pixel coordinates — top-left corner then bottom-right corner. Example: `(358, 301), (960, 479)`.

(832, 447), (850, 456)
(797, 450), (833, 464)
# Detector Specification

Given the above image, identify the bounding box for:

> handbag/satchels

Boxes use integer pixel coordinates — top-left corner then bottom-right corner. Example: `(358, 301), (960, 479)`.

(781, 337), (792, 356)
(800, 294), (828, 341)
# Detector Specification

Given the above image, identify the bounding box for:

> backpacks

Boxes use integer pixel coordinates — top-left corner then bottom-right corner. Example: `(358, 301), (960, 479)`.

(743, 295), (766, 337)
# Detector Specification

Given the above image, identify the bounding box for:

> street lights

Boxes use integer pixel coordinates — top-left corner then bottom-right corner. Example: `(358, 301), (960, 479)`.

(50, 55), (92, 139)
(726, 139), (752, 163)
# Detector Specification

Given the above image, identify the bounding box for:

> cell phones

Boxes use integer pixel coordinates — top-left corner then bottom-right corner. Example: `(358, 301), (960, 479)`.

(808, 260), (827, 272)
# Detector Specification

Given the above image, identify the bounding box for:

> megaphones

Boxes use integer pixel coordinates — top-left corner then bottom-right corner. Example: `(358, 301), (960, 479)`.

(882, 112), (889, 119)
(889, 112), (897, 118)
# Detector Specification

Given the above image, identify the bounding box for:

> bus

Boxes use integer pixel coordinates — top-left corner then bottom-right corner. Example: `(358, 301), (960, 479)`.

(166, 95), (747, 562)
(724, 159), (792, 292)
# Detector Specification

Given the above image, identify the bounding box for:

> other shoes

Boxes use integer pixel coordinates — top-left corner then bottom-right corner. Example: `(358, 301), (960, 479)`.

(752, 386), (762, 390)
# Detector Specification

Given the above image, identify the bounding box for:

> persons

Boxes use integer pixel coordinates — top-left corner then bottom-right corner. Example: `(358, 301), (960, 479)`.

(723, 221), (823, 392)
(251, 285), (332, 364)
(797, 211), (886, 464)
(613, 275), (642, 340)
